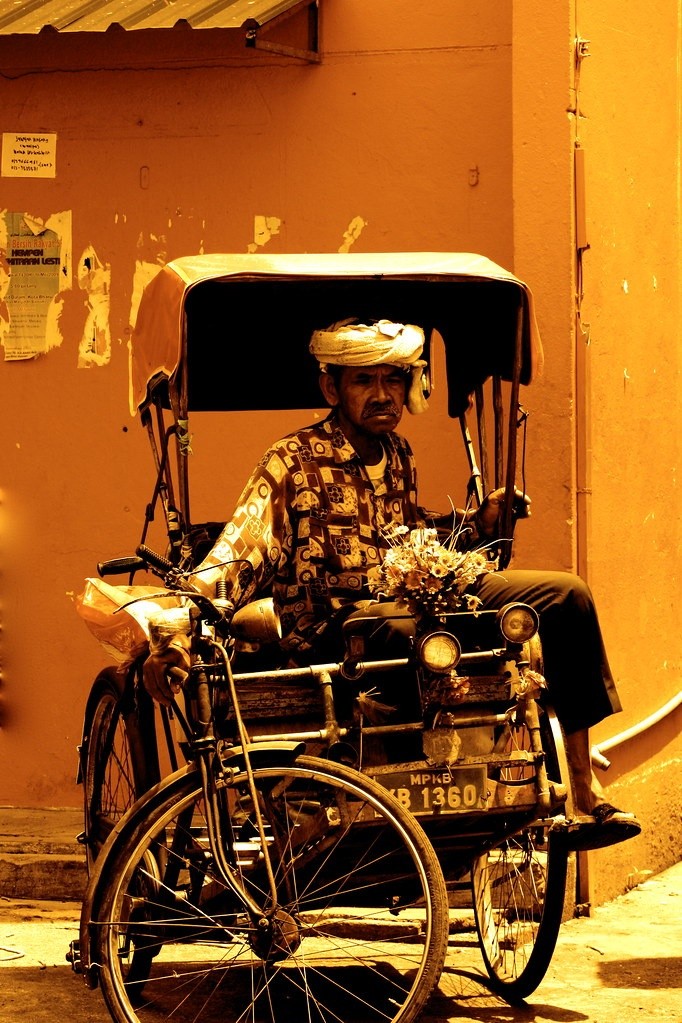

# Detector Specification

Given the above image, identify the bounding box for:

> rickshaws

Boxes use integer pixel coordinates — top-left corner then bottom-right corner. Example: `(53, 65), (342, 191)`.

(62, 248), (594, 1023)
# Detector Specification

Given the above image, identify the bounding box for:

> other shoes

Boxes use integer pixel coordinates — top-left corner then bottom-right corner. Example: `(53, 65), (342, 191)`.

(574, 802), (641, 851)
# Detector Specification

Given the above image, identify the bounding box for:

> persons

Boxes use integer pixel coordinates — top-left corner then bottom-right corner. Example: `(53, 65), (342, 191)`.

(143, 316), (641, 853)
